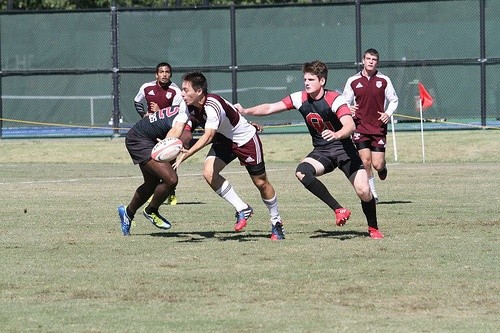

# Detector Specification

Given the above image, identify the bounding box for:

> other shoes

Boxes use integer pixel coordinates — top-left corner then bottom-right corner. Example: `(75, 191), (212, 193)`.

(375, 198), (379, 205)
(168, 191), (177, 206)
(147, 194), (154, 203)
(378, 168), (387, 181)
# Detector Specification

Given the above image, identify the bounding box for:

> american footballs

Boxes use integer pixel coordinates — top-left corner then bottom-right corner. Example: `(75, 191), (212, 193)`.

(150, 137), (183, 163)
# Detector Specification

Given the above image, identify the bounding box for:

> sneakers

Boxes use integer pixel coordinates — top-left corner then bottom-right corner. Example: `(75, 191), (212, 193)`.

(271, 219), (285, 240)
(234, 204), (254, 232)
(142, 206), (171, 229)
(368, 226), (383, 238)
(118, 205), (132, 235)
(334, 207), (351, 226)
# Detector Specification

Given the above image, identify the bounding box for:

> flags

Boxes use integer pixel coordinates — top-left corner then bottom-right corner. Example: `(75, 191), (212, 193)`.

(420, 84), (432, 106)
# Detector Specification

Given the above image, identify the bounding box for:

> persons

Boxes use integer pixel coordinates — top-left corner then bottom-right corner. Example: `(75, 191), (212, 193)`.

(118, 104), (263, 237)
(232, 60), (383, 239)
(343, 49), (399, 204)
(157, 72), (285, 240)
(134, 62), (186, 205)
